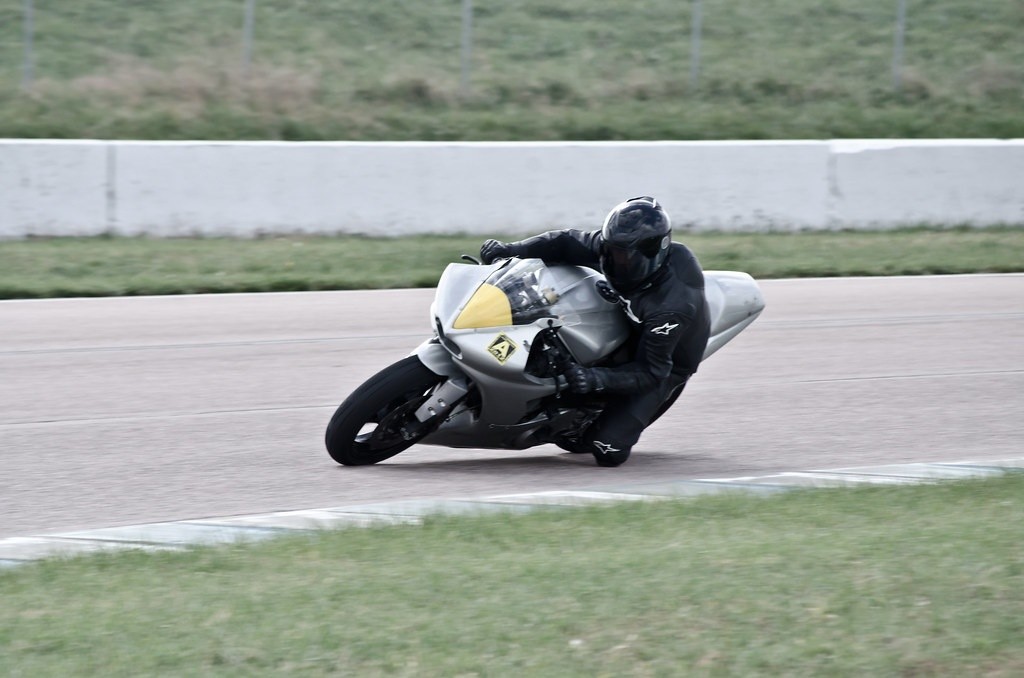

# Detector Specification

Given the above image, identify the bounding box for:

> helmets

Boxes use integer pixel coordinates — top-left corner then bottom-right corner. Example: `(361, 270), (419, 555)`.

(600, 196), (671, 293)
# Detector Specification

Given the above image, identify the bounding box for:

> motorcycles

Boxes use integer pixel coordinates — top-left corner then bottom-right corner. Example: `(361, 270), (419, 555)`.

(324, 256), (766, 468)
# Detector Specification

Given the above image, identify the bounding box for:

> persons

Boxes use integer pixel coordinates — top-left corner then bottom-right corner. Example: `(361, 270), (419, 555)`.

(480, 195), (712, 468)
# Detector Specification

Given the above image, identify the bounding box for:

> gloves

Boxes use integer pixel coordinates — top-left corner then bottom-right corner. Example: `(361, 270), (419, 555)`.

(481, 239), (507, 264)
(565, 363), (597, 394)
